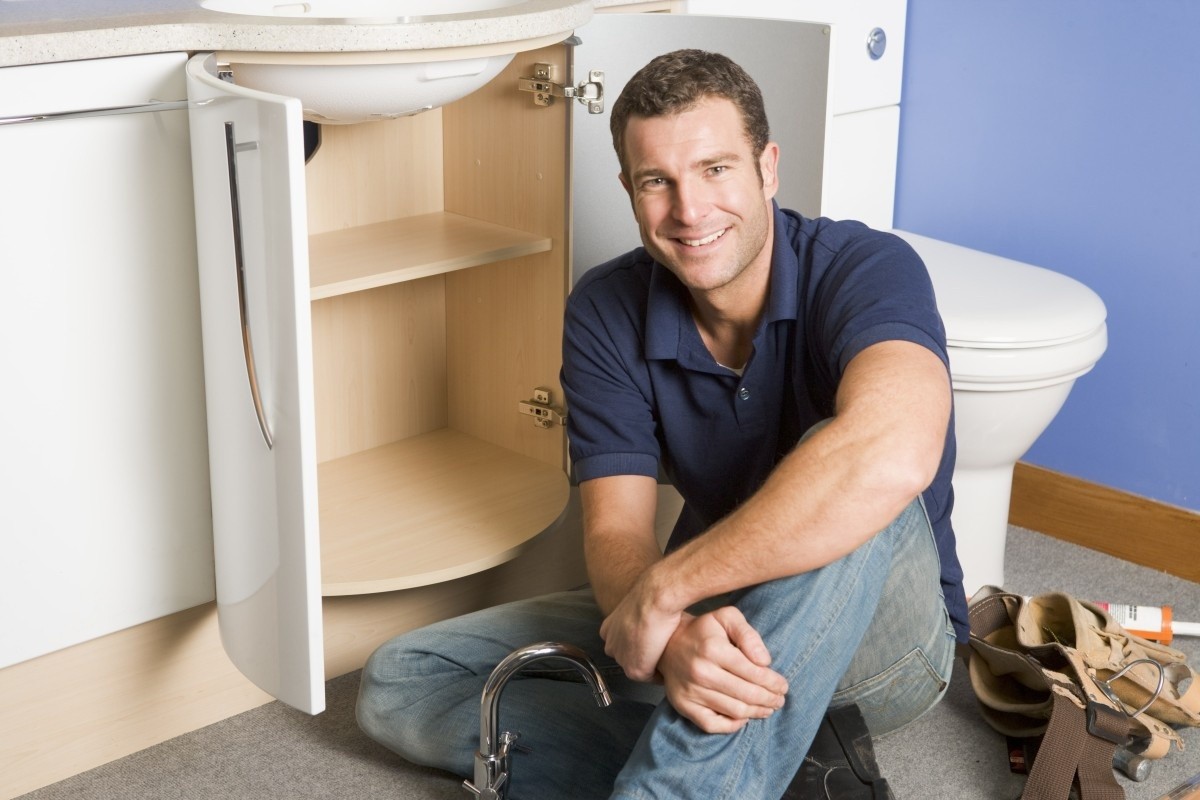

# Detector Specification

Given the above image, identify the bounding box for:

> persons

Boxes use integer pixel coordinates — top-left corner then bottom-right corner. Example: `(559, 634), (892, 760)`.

(354, 50), (969, 800)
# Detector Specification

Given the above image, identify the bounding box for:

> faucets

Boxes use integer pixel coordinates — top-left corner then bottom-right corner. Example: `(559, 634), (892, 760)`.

(461, 641), (615, 800)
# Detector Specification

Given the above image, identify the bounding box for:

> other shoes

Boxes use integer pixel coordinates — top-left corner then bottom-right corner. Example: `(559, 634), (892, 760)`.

(783, 704), (894, 800)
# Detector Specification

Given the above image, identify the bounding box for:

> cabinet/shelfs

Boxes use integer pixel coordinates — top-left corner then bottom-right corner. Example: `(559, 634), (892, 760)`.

(179, 37), (585, 713)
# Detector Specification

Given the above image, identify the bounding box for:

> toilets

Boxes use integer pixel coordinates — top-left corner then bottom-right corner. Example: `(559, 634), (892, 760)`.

(688, 0), (1110, 601)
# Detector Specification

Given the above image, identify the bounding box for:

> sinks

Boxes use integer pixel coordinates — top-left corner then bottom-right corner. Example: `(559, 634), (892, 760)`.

(200, 0), (596, 127)
